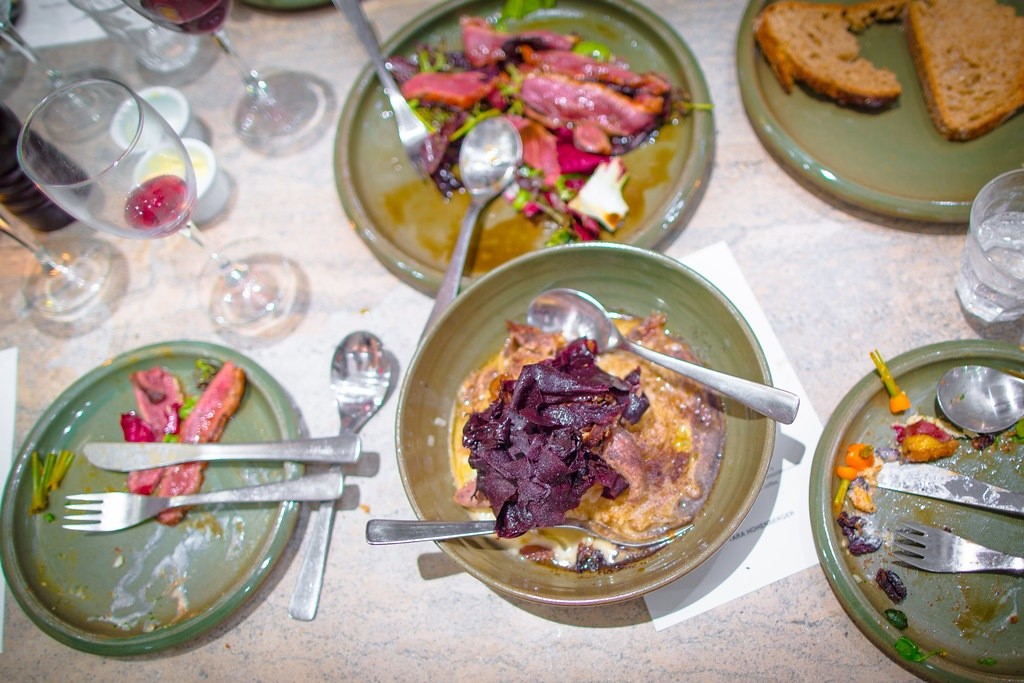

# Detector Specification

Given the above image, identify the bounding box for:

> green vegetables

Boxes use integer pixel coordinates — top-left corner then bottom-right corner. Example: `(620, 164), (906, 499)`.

(403, 0), (614, 248)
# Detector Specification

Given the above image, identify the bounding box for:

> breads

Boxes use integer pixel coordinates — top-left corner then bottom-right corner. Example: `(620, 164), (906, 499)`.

(750, 0), (1024, 139)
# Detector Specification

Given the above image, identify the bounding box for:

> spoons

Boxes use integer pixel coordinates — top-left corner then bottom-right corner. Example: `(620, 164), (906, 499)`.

(936, 365), (1024, 434)
(288, 330), (393, 620)
(419, 117), (524, 340)
(526, 289), (800, 425)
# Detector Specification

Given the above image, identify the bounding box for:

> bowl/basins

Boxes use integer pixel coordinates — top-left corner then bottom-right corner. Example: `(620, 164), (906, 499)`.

(396, 242), (776, 607)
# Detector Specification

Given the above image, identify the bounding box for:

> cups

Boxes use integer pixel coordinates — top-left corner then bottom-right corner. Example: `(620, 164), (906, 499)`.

(109, 87), (207, 156)
(955, 169), (1024, 323)
(134, 138), (232, 227)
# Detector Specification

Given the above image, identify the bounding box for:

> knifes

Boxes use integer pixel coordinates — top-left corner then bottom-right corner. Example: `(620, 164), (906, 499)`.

(863, 461), (1024, 516)
(80, 435), (362, 472)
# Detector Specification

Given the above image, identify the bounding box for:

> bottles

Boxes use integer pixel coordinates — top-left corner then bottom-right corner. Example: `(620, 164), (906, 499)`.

(0, 88), (102, 237)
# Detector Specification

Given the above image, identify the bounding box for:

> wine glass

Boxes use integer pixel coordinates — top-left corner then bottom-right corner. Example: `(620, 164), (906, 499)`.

(0, 0), (334, 340)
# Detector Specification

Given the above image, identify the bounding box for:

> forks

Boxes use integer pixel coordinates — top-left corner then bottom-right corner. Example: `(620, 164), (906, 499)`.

(62, 472), (342, 531)
(335, 1), (459, 188)
(892, 520), (1024, 576)
(365, 512), (696, 545)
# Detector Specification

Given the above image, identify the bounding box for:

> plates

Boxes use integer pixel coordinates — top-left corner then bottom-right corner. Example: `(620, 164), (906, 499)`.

(334, 1), (716, 302)
(738, 1), (1024, 223)
(808, 340), (1024, 683)
(0, 341), (304, 655)
